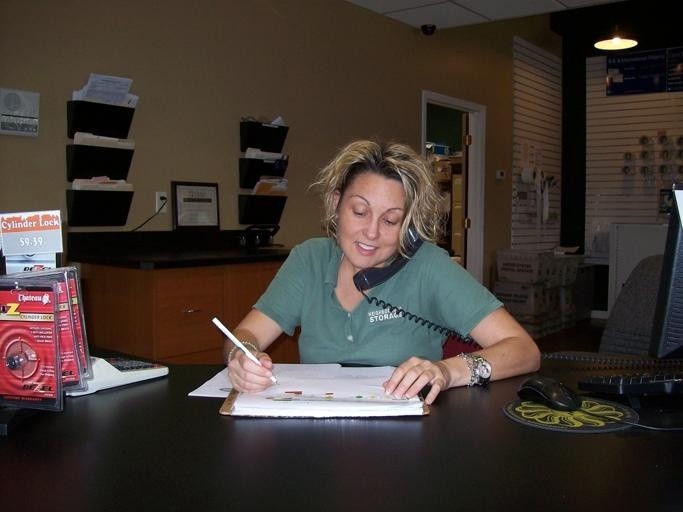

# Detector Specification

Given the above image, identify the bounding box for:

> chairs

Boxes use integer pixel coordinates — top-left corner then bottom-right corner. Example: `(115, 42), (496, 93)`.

(598, 253), (661, 355)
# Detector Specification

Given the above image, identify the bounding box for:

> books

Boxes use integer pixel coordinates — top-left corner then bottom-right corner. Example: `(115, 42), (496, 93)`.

(238, 113), (290, 198)
(187, 363), (431, 419)
(68, 72), (140, 192)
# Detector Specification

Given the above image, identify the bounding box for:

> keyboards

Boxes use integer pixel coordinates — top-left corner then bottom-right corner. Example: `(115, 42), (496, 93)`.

(578, 373), (683, 409)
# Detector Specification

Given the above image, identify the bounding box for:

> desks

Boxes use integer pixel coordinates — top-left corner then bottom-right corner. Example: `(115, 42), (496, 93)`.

(0, 344), (682, 510)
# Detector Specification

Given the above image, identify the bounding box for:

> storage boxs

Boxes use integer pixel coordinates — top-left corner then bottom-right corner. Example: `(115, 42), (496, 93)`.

(492, 246), (594, 339)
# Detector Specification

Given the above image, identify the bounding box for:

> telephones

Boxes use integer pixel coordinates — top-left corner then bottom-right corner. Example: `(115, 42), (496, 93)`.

(353, 221), (431, 292)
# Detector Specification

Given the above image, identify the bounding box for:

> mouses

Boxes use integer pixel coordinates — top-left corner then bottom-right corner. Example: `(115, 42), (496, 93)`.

(517, 376), (582, 411)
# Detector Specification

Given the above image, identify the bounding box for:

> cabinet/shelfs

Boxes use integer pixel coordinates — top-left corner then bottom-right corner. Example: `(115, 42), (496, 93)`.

(66, 260), (300, 362)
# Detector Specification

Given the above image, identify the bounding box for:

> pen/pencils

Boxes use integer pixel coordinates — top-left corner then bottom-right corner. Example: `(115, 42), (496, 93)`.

(212, 316), (278, 385)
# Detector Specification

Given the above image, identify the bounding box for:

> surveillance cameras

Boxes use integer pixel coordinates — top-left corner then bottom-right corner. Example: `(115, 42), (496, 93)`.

(421, 24), (436, 35)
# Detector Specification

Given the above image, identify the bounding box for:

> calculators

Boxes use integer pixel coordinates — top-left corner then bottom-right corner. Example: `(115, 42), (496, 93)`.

(66, 356), (169, 396)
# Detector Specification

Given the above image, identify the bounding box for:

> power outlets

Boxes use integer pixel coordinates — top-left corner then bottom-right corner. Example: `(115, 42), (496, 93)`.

(154, 191), (167, 215)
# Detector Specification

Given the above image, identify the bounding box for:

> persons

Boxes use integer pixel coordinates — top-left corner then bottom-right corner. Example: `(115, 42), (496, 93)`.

(223, 140), (543, 406)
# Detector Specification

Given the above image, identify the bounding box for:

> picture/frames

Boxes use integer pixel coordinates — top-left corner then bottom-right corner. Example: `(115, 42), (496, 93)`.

(169, 180), (220, 232)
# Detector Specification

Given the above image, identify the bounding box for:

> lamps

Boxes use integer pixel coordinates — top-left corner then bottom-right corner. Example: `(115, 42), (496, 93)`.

(587, 24), (637, 53)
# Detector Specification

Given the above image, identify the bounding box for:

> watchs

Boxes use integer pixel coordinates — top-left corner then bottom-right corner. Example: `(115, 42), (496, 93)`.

(457, 351), (492, 389)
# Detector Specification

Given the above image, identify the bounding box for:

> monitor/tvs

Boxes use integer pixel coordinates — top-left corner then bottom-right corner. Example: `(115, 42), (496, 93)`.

(650, 181), (683, 358)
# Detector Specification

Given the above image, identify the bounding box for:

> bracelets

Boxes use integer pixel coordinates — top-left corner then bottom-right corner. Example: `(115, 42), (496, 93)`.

(225, 340), (260, 362)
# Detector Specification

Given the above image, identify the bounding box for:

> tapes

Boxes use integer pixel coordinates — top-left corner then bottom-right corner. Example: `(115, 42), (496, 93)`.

(495, 169), (506, 180)
(522, 166), (546, 184)
